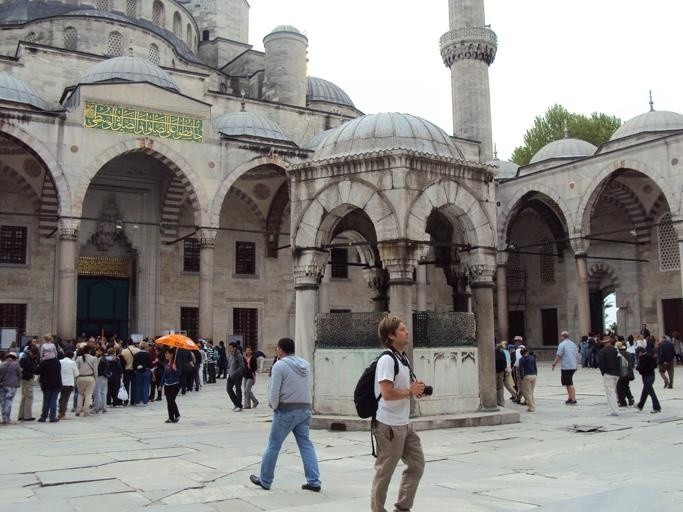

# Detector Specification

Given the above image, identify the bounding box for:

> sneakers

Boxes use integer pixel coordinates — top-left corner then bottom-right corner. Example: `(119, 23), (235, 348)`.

(25, 417), (35, 421)
(36, 418), (46, 422)
(90, 409), (98, 414)
(102, 407), (107, 414)
(116, 405), (123, 408)
(565, 399), (577, 404)
(107, 404), (113, 408)
(252, 400), (259, 408)
(165, 418), (175, 423)
(629, 398), (634, 406)
(663, 382), (668, 387)
(393, 505), (410, 512)
(142, 404), (149, 407)
(17, 418), (24, 420)
(50, 416), (59, 423)
(175, 414), (182, 423)
(235, 407), (243, 412)
(134, 403), (141, 406)
(84, 412), (89, 416)
(75, 412), (80, 416)
(243, 406), (251, 409)
(216, 375), (220, 378)
(608, 412), (619, 416)
(232, 405), (236, 410)
(669, 386), (673, 389)
(617, 404), (627, 407)
(223, 375), (226, 378)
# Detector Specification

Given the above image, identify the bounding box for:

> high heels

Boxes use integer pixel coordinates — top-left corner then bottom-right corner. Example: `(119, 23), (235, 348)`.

(651, 409), (661, 413)
(636, 404), (641, 410)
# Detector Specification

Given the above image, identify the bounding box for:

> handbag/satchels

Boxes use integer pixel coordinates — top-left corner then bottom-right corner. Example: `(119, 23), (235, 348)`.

(252, 373), (256, 385)
(619, 367), (634, 380)
(117, 386), (128, 400)
(103, 363), (112, 378)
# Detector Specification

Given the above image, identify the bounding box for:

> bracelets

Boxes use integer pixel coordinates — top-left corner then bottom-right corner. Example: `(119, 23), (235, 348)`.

(552, 364), (555, 366)
(405, 388), (413, 399)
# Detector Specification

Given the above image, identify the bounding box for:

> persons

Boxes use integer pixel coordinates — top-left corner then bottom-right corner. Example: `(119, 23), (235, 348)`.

(495, 335), (537, 412)
(551, 331), (582, 404)
(579, 323), (683, 413)
(272, 347), (280, 366)
(0, 329), (258, 426)
(371, 314), (427, 512)
(250, 337), (321, 491)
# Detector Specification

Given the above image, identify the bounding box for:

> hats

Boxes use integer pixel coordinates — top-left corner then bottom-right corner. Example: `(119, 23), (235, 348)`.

(600, 336), (613, 343)
(5, 351), (17, 359)
(613, 341), (627, 349)
(514, 336), (522, 341)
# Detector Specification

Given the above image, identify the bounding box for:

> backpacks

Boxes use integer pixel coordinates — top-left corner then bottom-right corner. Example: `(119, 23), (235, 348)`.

(353, 351), (398, 419)
(209, 346), (219, 360)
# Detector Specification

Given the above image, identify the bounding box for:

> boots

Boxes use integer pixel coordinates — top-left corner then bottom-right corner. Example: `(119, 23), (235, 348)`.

(149, 390), (155, 402)
(156, 391), (162, 401)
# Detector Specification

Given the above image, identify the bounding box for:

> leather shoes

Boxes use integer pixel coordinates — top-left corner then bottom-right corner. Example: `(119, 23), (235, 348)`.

(250, 474), (269, 489)
(302, 483), (321, 492)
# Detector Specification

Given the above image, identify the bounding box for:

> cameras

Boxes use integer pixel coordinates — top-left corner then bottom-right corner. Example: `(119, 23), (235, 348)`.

(413, 383), (437, 395)
